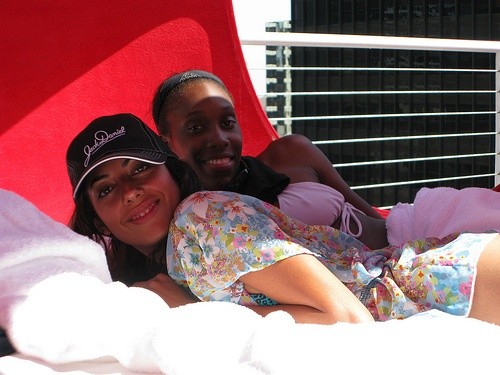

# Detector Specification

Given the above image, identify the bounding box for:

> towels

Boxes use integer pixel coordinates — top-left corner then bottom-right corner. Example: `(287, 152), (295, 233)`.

(1, 189), (500, 375)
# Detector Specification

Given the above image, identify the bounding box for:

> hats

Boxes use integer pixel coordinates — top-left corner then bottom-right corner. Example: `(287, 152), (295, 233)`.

(66, 114), (171, 198)
